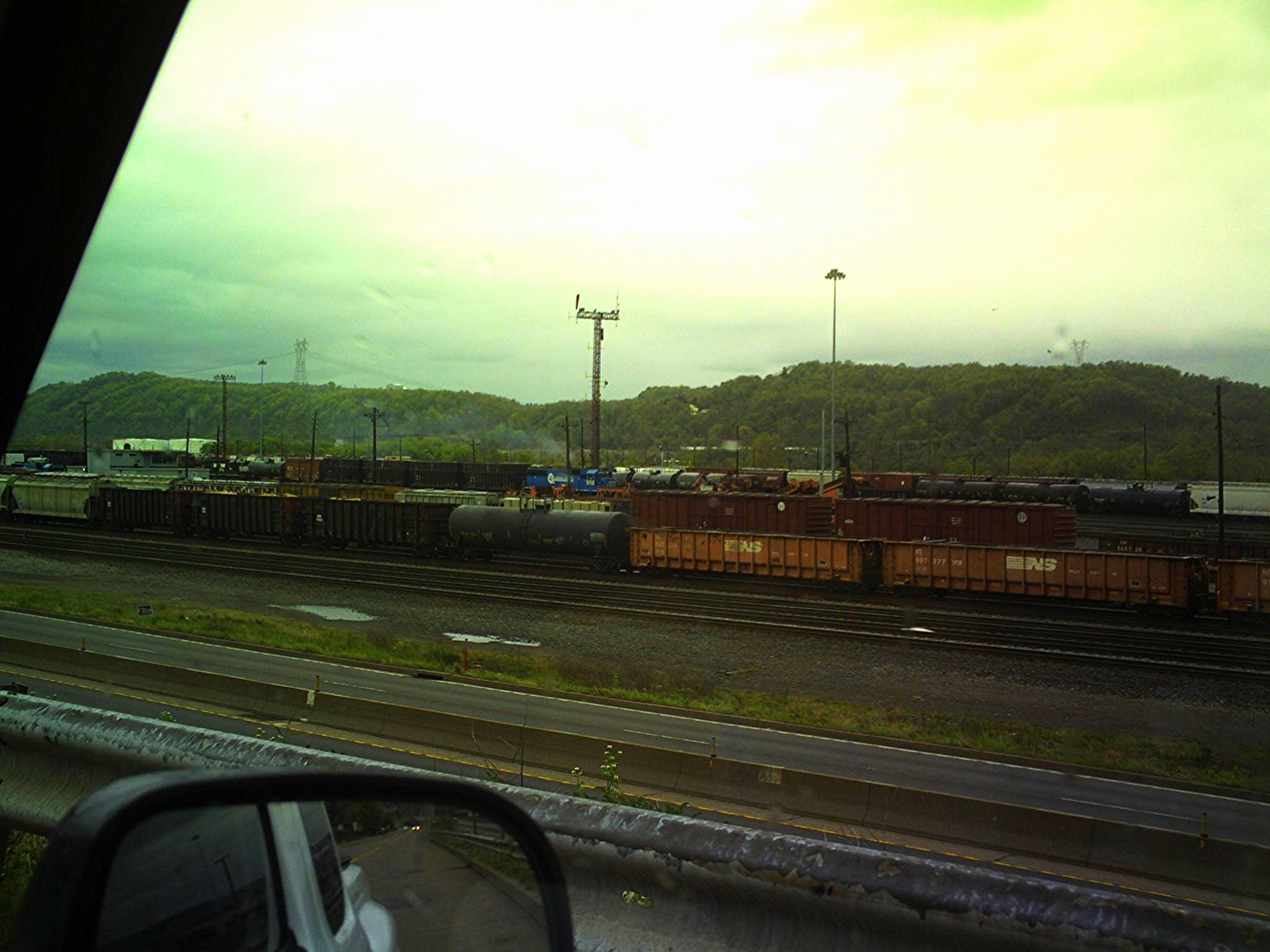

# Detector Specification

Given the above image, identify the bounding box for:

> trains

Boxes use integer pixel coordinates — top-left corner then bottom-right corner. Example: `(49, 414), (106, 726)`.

(0, 453), (1270, 623)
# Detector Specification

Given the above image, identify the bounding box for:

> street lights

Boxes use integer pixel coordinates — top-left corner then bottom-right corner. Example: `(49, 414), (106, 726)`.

(824, 268), (847, 477)
(258, 359), (269, 459)
